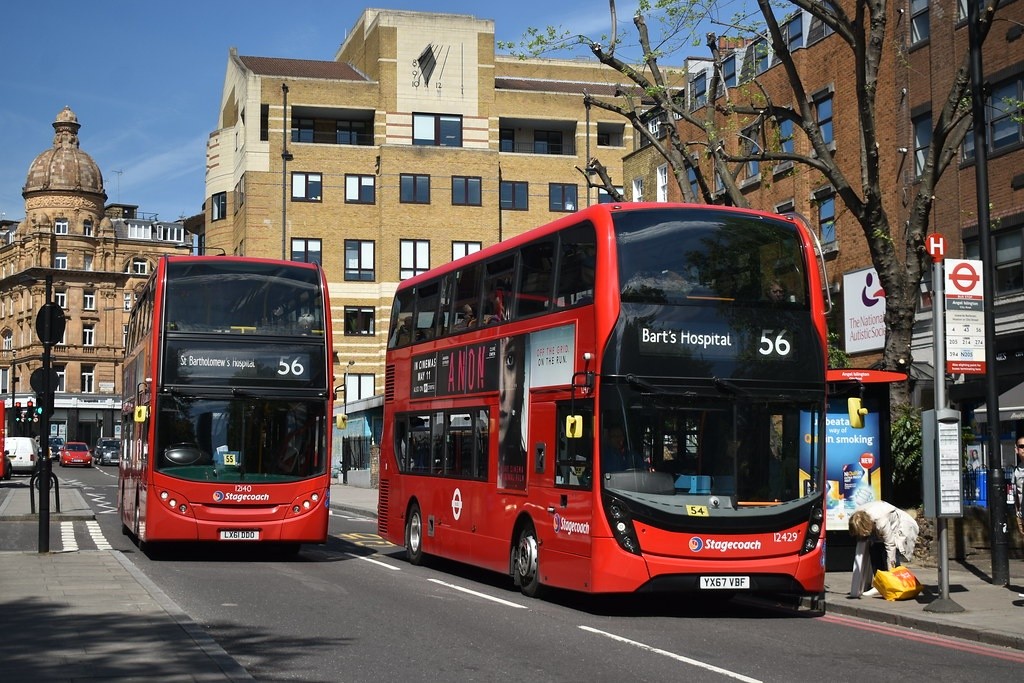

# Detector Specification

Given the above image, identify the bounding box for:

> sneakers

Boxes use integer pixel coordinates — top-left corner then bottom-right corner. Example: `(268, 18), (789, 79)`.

(863, 587), (882, 598)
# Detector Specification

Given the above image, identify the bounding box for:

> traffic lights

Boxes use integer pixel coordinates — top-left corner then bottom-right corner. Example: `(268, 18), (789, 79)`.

(36, 393), (42, 415)
(26, 401), (34, 423)
(15, 402), (21, 423)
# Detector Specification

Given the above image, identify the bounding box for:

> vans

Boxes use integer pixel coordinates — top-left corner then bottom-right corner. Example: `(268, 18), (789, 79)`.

(4, 437), (40, 477)
(94, 437), (121, 467)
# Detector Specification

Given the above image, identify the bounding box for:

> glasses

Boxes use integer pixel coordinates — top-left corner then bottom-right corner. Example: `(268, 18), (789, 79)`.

(1017, 444), (1024, 449)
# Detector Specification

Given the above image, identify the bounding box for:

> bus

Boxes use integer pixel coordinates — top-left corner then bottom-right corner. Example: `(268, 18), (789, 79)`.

(115, 255), (348, 555)
(377, 202), (868, 608)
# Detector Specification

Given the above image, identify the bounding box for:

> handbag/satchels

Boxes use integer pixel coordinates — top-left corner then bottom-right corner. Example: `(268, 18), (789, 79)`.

(873, 566), (923, 603)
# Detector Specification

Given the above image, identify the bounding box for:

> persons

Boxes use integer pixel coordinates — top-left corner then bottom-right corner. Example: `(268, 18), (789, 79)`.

(849, 500), (919, 596)
(396, 286), (509, 346)
(499, 334), (527, 491)
(1012, 438), (1024, 607)
(604, 423), (655, 473)
(972, 450), (979, 471)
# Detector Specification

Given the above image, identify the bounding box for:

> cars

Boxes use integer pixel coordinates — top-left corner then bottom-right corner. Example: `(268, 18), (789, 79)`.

(59, 442), (93, 468)
(35, 435), (65, 462)
